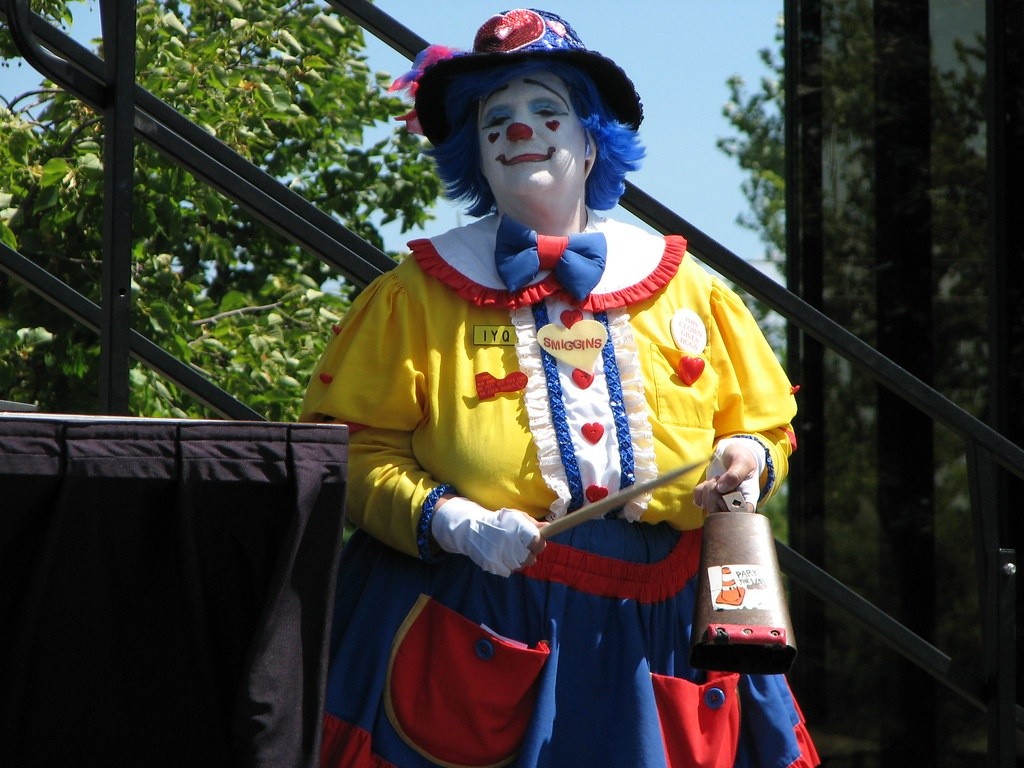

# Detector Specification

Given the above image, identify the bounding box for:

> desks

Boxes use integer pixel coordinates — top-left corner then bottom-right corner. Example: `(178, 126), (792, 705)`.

(0, 412), (350, 768)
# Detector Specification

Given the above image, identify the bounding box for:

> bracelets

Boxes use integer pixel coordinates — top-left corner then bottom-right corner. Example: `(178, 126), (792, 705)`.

(733, 435), (775, 502)
(416, 483), (455, 564)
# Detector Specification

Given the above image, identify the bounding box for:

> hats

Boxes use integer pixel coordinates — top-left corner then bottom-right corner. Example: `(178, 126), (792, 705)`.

(387, 8), (645, 148)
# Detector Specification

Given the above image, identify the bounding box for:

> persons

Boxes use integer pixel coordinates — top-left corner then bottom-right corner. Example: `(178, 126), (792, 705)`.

(296, 8), (822, 768)
